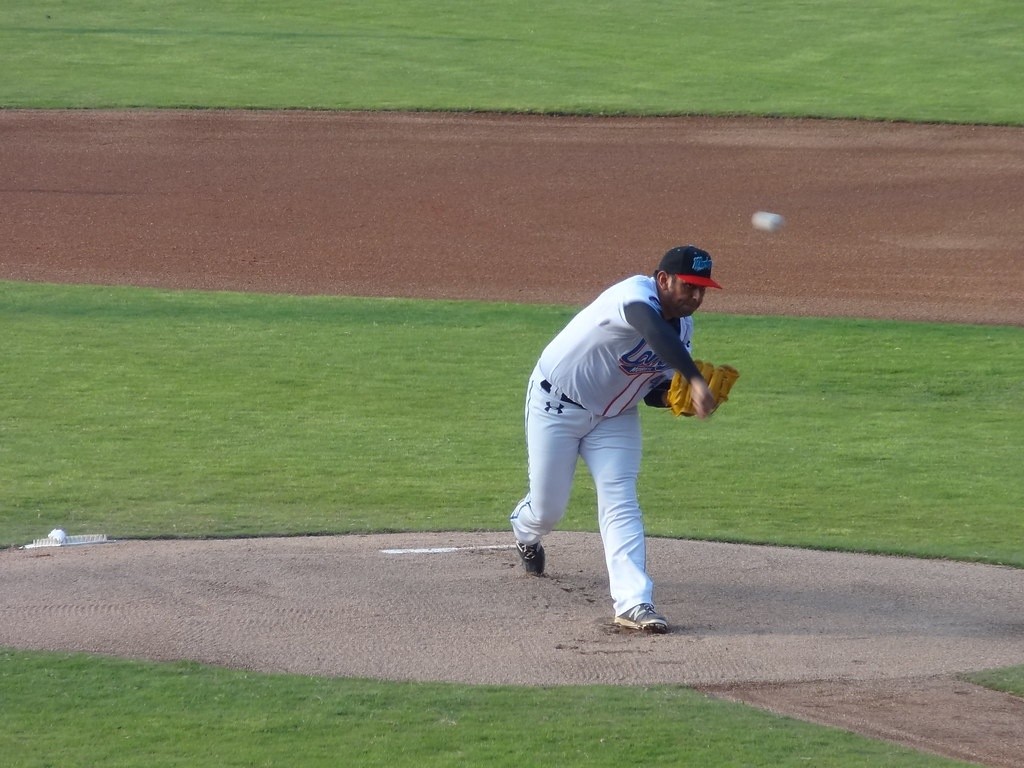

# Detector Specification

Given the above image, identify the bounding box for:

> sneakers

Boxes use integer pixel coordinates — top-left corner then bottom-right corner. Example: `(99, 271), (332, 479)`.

(615, 602), (667, 628)
(514, 539), (545, 574)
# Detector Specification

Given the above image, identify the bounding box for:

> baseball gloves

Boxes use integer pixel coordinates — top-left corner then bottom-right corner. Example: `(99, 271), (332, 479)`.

(667, 359), (741, 418)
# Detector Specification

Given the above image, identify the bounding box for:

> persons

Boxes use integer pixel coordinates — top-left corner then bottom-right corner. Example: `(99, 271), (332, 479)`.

(511, 247), (738, 634)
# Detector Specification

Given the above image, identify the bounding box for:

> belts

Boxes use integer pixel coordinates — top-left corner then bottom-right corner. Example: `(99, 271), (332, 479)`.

(541, 379), (586, 409)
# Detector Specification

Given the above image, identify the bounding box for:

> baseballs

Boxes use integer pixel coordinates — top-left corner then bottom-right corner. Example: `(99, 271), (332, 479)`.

(750, 211), (786, 233)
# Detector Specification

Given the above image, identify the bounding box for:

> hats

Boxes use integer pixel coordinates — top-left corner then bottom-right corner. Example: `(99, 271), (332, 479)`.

(661, 245), (723, 289)
